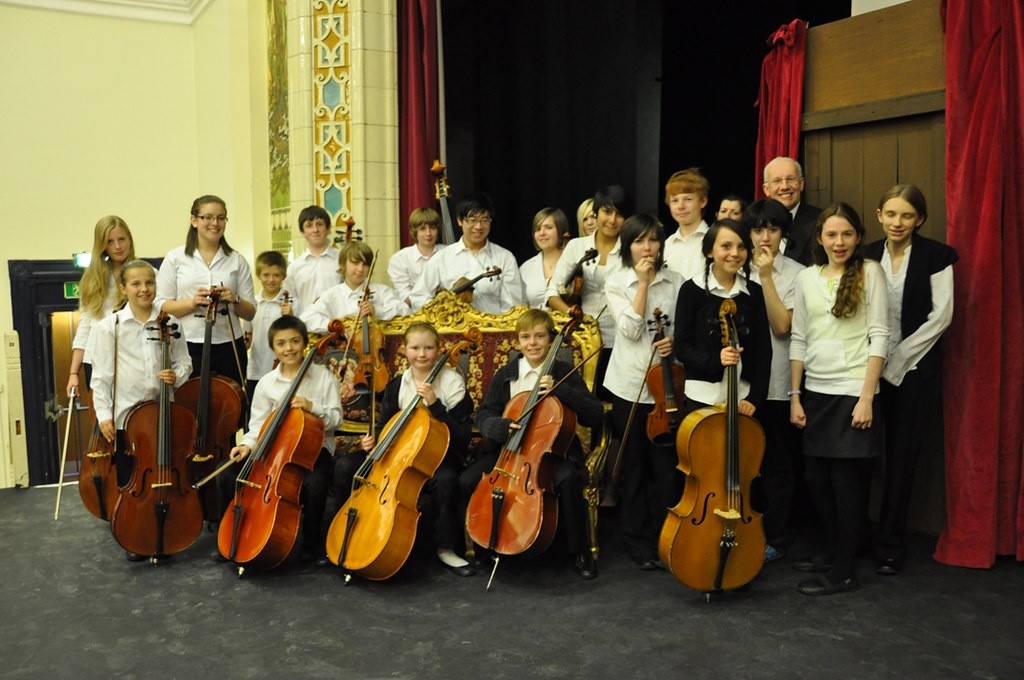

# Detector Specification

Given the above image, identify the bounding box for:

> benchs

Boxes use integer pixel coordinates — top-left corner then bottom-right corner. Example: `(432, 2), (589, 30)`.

(303, 290), (614, 561)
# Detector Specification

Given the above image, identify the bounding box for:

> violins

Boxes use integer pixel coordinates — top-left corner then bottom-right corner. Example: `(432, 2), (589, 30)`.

(546, 248), (598, 305)
(436, 266), (501, 303)
(270, 290), (294, 369)
(314, 217), (363, 304)
(350, 287), (389, 393)
(647, 306), (685, 449)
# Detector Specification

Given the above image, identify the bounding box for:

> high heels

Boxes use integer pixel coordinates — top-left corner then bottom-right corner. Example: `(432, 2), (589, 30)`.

(126, 550), (148, 562)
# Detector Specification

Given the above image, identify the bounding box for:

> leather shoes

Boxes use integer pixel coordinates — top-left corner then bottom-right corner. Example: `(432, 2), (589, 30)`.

(433, 548), (475, 576)
(469, 548), (494, 570)
(792, 550), (836, 573)
(852, 554), (904, 574)
(797, 572), (860, 596)
(571, 547), (598, 578)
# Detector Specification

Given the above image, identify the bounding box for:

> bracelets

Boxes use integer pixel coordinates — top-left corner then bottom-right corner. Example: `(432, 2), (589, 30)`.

(787, 389), (802, 398)
(69, 372), (79, 375)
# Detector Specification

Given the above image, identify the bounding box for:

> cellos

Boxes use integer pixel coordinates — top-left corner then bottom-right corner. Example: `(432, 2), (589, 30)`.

(657, 299), (767, 605)
(325, 325), (484, 586)
(109, 310), (203, 565)
(214, 318), (345, 579)
(431, 160), (456, 245)
(173, 285), (248, 523)
(465, 304), (585, 592)
(78, 387), (118, 521)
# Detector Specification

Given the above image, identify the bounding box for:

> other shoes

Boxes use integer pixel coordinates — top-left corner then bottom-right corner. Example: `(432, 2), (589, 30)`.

(636, 545), (658, 569)
(211, 548), (229, 563)
(316, 551), (331, 568)
(762, 545), (785, 564)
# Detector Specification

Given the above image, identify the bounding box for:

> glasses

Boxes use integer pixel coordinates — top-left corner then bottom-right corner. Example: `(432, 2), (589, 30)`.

(768, 176), (801, 186)
(195, 215), (229, 225)
(463, 216), (492, 226)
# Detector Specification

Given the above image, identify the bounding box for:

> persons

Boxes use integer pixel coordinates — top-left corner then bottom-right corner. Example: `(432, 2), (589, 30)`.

(332, 321), (475, 576)
(788, 201), (890, 597)
(743, 201), (810, 561)
(575, 198), (601, 239)
(455, 310), (614, 579)
(152, 195), (258, 531)
(240, 156), (823, 566)
(94, 264), (192, 560)
(212, 316), (344, 563)
(67, 215), (159, 560)
(860, 183), (959, 575)
(604, 211), (690, 570)
(672, 218), (778, 562)
